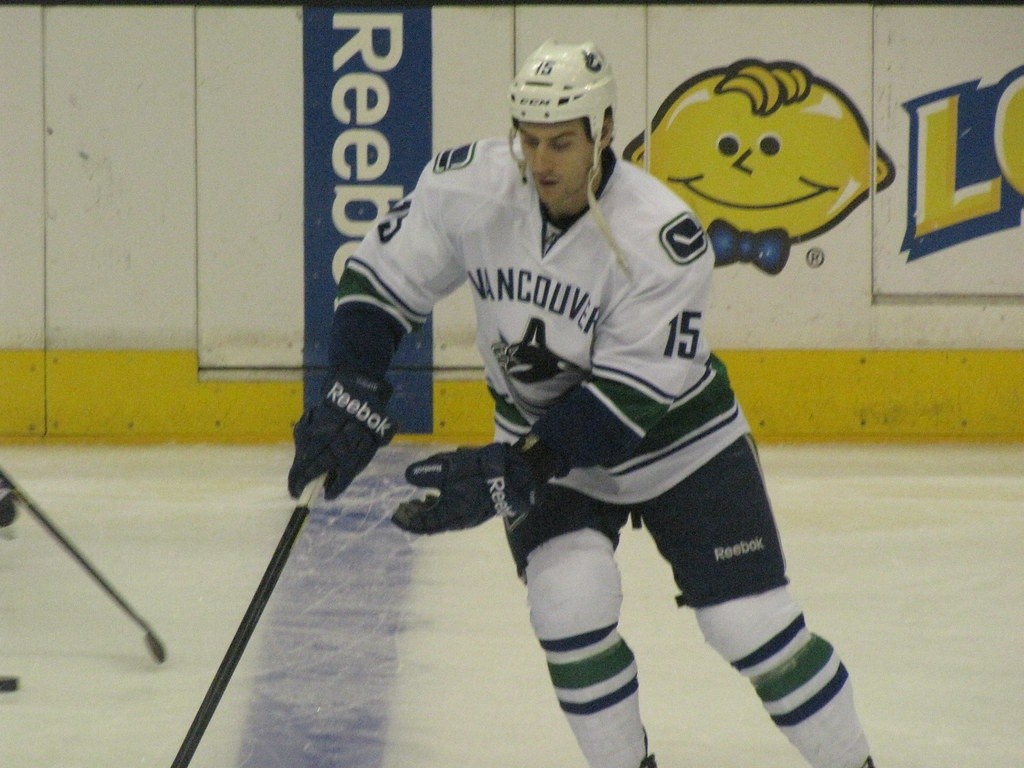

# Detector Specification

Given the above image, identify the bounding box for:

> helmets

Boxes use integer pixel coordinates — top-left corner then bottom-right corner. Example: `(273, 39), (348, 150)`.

(507, 41), (618, 142)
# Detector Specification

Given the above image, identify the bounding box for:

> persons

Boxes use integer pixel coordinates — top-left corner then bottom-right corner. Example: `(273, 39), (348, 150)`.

(0, 477), (21, 692)
(286, 40), (874, 767)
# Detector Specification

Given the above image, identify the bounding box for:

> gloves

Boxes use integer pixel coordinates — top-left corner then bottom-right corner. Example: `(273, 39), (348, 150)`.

(287, 365), (401, 500)
(390, 442), (544, 535)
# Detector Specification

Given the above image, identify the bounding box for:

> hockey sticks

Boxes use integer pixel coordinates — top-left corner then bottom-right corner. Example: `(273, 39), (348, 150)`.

(170, 471), (326, 768)
(1, 464), (167, 667)
